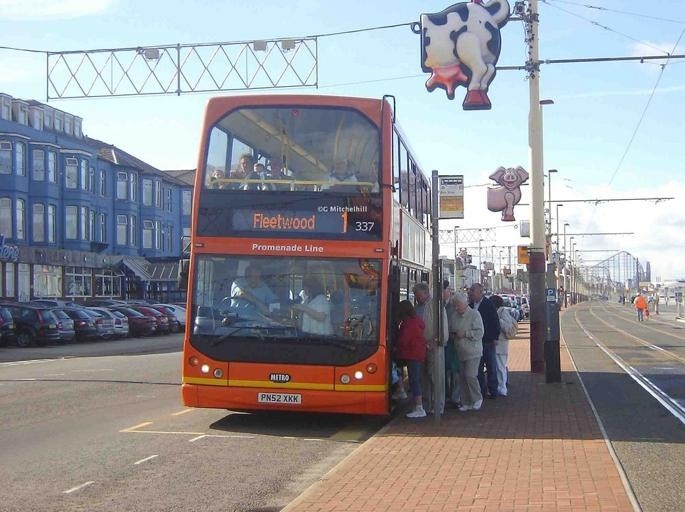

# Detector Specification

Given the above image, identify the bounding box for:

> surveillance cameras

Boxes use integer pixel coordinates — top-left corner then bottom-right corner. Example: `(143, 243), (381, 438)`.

(514, 0), (529, 15)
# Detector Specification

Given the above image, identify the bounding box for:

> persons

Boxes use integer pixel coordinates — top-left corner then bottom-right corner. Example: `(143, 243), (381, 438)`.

(230, 263), (280, 317)
(321, 157), (357, 190)
(211, 153), (292, 192)
(292, 273), (335, 335)
(392, 282), (512, 419)
(619, 292), (656, 321)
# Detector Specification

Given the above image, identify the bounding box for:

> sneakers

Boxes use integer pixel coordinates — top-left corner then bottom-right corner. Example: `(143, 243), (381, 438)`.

(406, 410), (427, 417)
(481, 389), (508, 399)
(446, 397), (483, 411)
(390, 389), (409, 399)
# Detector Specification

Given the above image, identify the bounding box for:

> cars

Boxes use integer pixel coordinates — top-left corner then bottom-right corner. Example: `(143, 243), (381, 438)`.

(0, 297), (186, 349)
(489, 289), (586, 326)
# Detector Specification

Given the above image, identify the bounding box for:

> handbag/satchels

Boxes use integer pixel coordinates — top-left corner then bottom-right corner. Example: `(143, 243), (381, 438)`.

(504, 316), (519, 339)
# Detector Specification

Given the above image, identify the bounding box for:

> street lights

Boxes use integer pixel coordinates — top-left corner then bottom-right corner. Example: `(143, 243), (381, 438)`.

(529, 96), (592, 389)
(445, 223), (526, 296)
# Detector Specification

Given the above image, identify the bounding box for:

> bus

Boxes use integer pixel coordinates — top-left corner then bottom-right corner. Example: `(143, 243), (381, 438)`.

(177, 89), (438, 425)
